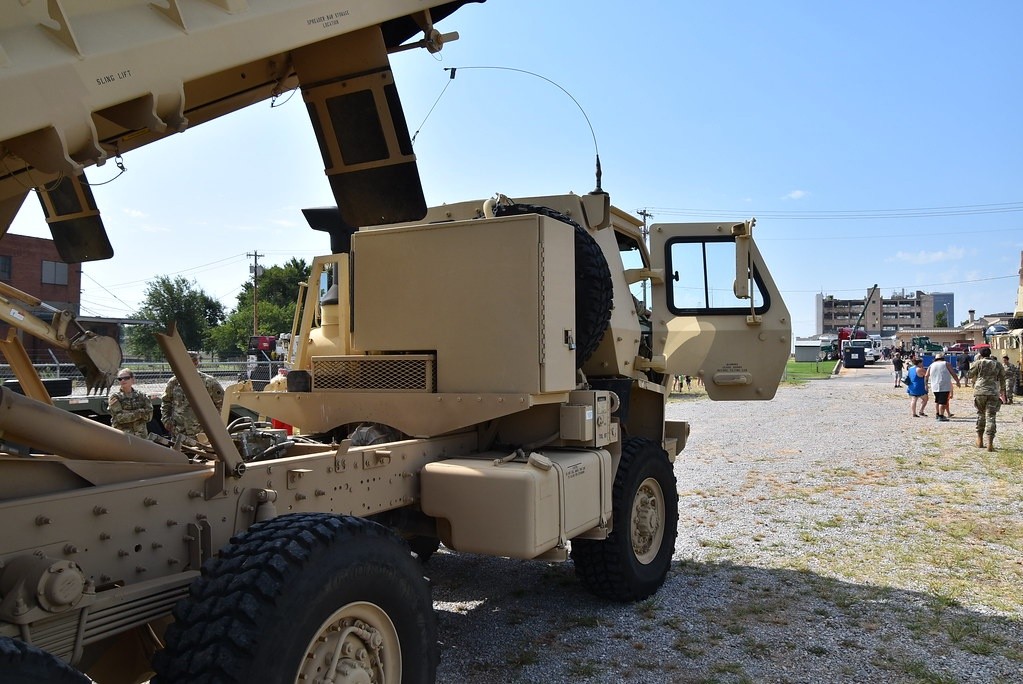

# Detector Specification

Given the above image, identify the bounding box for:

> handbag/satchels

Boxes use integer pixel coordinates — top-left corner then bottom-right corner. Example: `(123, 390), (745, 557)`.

(901, 376), (910, 385)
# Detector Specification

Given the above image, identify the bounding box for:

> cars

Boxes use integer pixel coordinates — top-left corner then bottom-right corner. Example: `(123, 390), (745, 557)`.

(947, 343), (971, 351)
(986, 326), (1010, 335)
(970, 344), (991, 351)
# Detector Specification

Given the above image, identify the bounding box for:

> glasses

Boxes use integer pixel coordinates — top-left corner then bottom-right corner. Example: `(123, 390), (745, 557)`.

(117, 376), (132, 381)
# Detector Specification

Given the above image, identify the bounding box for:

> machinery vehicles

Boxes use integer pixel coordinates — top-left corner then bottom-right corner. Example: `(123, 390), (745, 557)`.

(0, 1), (791, 684)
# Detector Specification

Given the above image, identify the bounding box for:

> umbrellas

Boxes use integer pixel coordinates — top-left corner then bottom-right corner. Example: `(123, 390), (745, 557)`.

(971, 344), (990, 349)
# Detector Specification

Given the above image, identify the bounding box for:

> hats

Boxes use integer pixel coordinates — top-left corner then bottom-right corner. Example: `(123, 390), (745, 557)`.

(188, 351), (198, 361)
(935, 354), (943, 360)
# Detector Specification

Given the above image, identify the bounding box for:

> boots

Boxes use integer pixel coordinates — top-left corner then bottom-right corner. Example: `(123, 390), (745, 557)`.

(987, 434), (994, 452)
(976, 431), (984, 447)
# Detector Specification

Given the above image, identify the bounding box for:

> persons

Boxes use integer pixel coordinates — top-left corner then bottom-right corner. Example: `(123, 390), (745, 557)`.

(161, 352), (224, 441)
(108, 369), (153, 440)
(956, 349), (972, 387)
(892, 353), (905, 388)
(925, 354), (961, 421)
(991, 356), (1018, 404)
(882, 345), (915, 360)
(673, 375), (703, 392)
(966, 347), (1006, 451)
(906, 355), (928, 418)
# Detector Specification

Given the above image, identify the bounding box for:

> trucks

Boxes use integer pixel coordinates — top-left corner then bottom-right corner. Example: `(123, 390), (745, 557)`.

(838, 328), (883, 368)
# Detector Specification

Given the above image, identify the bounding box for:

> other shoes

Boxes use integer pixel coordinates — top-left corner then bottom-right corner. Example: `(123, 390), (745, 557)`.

(1008, 399), (1013, 404)
(913, 414), (920, 417)
(939, 415), (950, 421)
(936, 415), (939, 419)
(965, 385), (969, 387)
(1004, 399), (1007, 404)
(919, 412), (928, 416)
(898, 385), (902, 387)
(948, 414), (954, 418)
(895, 385), (897, 388)
